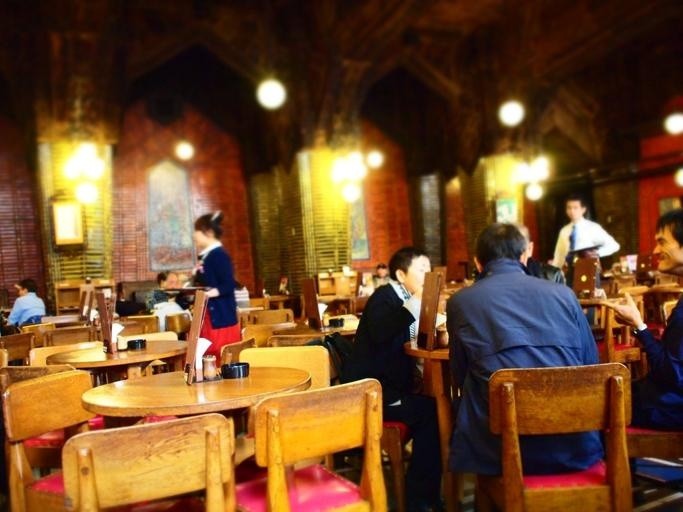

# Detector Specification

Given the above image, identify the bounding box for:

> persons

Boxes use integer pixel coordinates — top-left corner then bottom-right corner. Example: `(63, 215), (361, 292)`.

(4, 279), (46, 366)
(554, 193), (621, 323)
(475, 222), (566, 285)
(94, 212), (242, 369)
(445, 222), (604, 473)
(370, 262), (388, 288)
(599, 209), (681, 505)
(348, 243), (448, 511)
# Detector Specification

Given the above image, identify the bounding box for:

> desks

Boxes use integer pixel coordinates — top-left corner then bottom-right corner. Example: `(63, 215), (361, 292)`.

(405, 340), (454, 509)
(81, 367), (311, 414)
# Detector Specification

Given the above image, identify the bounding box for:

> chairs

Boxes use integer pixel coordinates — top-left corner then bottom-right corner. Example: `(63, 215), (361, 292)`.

(490, 363), (635, 511)
(321, 335), (408, 509)
(3, 368), (94, 512)
(62, 413), (237, 511)
(2, 254), (683, 372)
(235, 379), (388, 511)
(235, 346), (336, 478)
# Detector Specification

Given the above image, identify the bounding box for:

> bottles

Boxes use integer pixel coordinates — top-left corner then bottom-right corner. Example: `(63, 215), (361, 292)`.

(436, 327), (449, 348)
(201, 354), (217, 381)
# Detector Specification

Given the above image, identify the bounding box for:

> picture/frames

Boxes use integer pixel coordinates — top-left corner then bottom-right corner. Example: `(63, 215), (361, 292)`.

(50, 189), (88, 259)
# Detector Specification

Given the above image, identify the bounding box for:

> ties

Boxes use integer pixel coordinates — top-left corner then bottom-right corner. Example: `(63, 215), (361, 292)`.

(569, 225), (575, 248)
